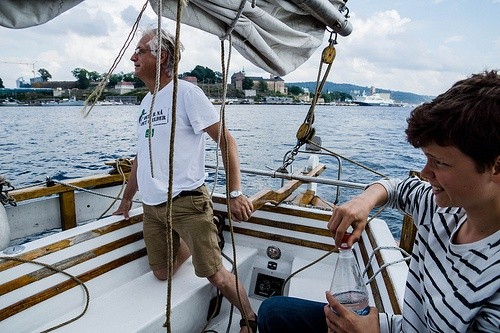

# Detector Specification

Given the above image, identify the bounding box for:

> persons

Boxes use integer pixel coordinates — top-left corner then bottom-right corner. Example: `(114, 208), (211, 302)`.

(113, 28), (257, 333)
(257, 69), (500, 333)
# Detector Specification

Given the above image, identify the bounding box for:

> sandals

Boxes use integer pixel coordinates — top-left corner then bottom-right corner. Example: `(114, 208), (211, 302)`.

(212, 213), (225, 250)
(240, 312), (258, 333)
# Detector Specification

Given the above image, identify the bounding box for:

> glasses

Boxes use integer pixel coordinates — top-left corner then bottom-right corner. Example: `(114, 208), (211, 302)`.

(134, 47), (157, 54)
(155, 189), (203, 206)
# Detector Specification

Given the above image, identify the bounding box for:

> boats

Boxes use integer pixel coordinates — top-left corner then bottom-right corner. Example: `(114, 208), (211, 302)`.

(351, 95), (392, 105)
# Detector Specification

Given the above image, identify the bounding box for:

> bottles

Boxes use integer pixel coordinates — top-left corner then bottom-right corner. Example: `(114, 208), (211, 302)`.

(329, 235), (370, 317)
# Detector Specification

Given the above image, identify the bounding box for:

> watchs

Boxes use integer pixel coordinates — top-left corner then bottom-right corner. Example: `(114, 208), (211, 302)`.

(229, 190), (243, 200)
